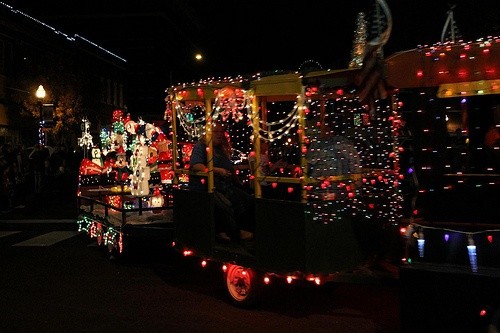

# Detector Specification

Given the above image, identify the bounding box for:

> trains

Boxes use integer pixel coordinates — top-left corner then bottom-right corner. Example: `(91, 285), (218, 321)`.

(75, 38), (500, 306)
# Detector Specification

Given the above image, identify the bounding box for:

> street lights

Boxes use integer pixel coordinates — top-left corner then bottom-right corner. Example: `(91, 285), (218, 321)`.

(33, 83), (54, 193)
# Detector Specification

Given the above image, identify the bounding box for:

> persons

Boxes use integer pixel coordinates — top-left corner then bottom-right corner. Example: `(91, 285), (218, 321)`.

(189, 123), (254, 242)
